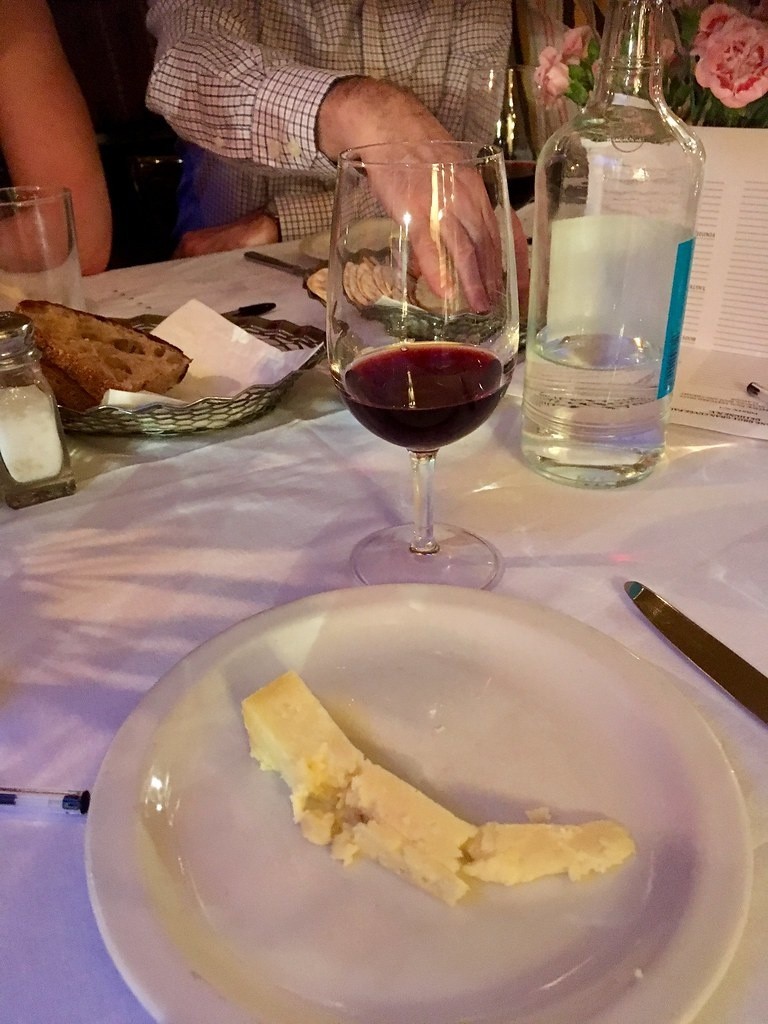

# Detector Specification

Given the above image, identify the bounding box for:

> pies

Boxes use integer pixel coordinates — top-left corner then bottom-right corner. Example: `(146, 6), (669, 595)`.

(307, 238), (494, 318)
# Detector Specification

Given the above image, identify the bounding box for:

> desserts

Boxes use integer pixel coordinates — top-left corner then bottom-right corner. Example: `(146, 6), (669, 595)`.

(240, 671), (635, 906)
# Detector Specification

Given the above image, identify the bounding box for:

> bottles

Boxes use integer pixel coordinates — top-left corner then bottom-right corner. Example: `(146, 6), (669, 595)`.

(0, 311), (76, 510)
(520, 0), (704, 488)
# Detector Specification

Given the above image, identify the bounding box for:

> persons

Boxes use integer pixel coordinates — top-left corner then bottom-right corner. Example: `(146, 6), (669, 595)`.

(0, 1), (707, 315)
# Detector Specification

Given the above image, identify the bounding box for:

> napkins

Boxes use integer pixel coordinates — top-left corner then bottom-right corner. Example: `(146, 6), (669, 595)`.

(96, 296), (325, 428)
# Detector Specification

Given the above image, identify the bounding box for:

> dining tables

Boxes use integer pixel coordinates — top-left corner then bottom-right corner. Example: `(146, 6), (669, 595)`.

(0, 204), (768, 1024)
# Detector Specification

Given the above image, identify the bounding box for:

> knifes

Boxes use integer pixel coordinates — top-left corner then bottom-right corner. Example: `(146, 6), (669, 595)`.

(624, 583), (767, 732)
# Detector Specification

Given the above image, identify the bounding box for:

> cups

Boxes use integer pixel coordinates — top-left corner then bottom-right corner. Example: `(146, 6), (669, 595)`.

(1, 184), (88, 314)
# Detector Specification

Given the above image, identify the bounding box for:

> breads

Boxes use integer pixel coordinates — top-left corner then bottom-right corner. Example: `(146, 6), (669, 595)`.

(13, 298), (191, 410)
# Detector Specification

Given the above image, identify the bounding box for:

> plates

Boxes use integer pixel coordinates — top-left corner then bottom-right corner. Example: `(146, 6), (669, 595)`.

(83, 581), (754, 1024)
(296, 217), (400, 261)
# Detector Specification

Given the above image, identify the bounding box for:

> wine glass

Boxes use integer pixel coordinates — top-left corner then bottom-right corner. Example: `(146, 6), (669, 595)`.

(324, 140), (518, 593)
(462, 65), (547, 213)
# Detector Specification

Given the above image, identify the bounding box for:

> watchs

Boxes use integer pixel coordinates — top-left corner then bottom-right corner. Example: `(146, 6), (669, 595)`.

(262, 198), (281, 242)
